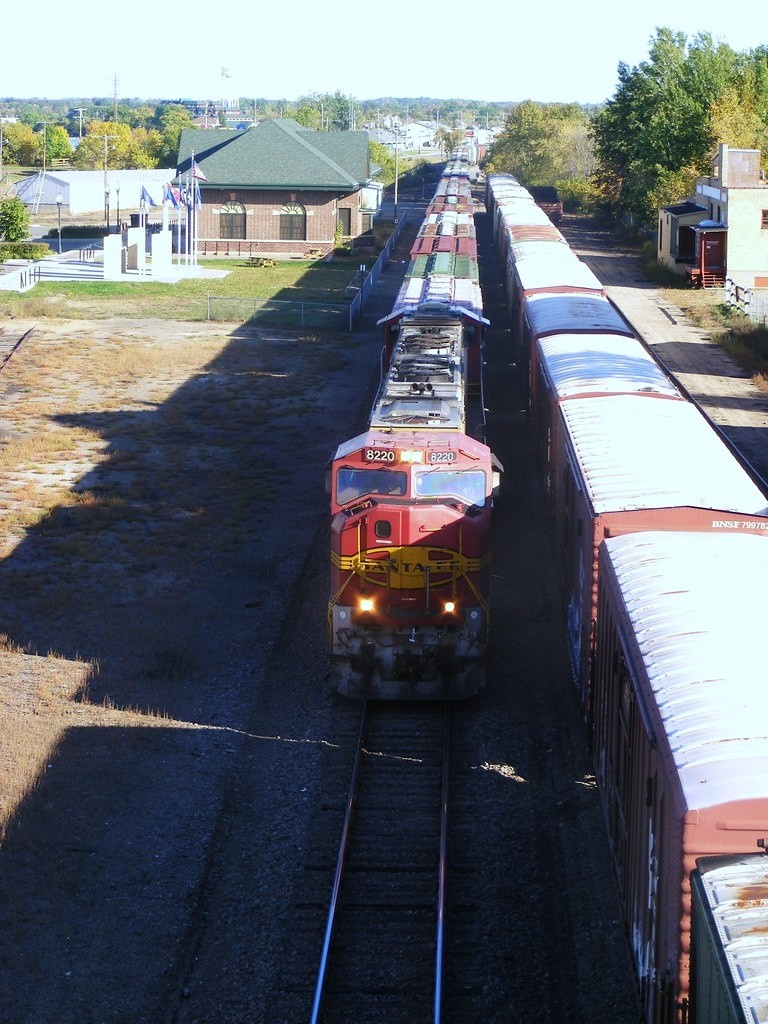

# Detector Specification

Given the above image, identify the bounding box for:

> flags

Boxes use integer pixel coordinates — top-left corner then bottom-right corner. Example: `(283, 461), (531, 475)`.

(141, 186), (157, 206)
(162, 180), (202, 211)
(193, 160), (209, 182)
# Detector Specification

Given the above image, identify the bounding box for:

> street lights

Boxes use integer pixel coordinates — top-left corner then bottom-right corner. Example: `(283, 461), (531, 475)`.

(115, 181), (120, 230)
(105, 184), (111, 235)
(55, 191), (64, 253)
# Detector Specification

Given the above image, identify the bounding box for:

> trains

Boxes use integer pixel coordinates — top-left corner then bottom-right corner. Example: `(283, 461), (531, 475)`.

(479, 174), (768, 1017)
(326, 128), (505, 708)
